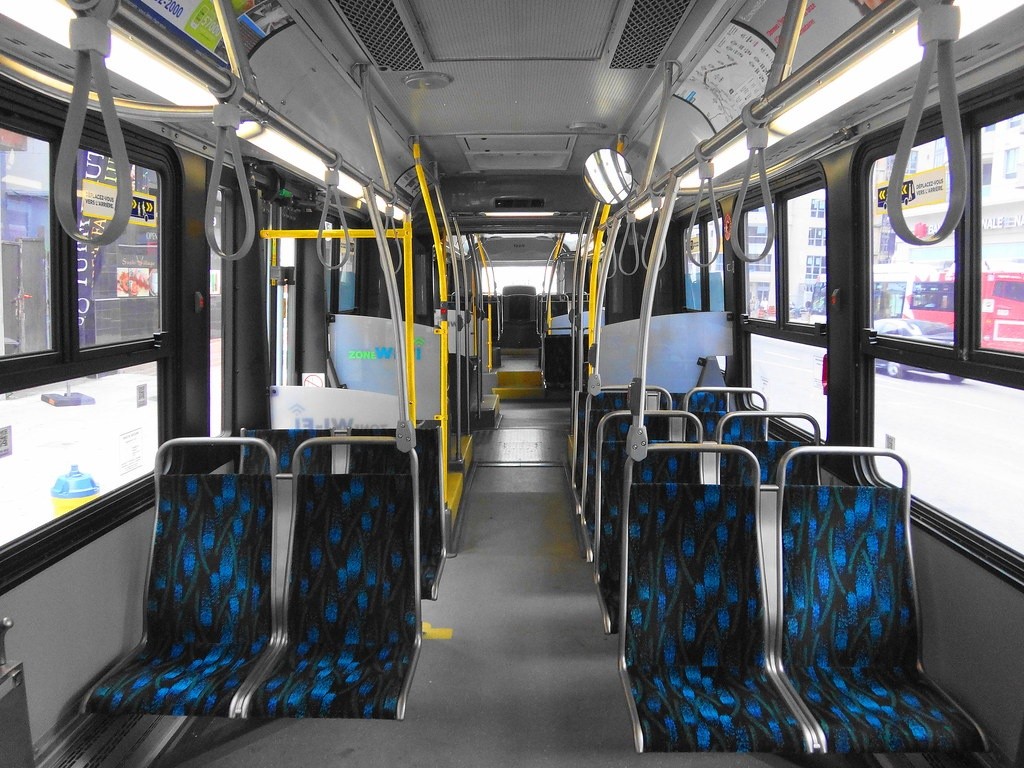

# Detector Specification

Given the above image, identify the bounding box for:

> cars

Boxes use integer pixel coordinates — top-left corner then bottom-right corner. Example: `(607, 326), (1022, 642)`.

(875, 319), (964, 382)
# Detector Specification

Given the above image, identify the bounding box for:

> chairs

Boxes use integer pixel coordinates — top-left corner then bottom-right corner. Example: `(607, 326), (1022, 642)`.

(571, 387), (987, 768)
(434, 285), (588, 439)
(78, 423), (441, 722)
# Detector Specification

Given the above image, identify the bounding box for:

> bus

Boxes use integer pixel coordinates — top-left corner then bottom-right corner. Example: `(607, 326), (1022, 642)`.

(805, 269), (1024, 353)
